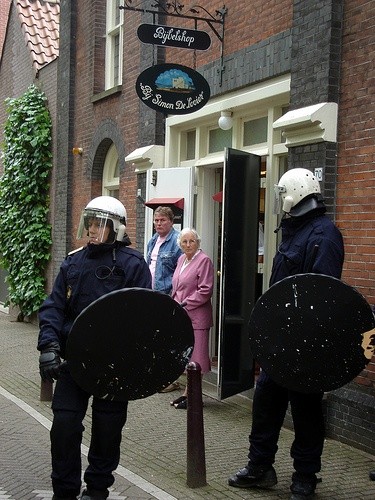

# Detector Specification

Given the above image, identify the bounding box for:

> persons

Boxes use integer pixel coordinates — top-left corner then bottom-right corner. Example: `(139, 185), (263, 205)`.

(145, 206), (214, 409)
(36, 196), (153, 500)
(229, 168), (343, 500)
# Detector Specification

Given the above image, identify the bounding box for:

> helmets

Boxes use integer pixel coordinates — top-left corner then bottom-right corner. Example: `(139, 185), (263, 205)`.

(83, 195), (126, 242)
(278, 166), (321, 213)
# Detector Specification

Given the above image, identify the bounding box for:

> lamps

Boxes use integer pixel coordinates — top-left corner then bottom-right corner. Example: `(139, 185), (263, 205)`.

(218, 112), (233, 130)
(72, 147), (83, 155)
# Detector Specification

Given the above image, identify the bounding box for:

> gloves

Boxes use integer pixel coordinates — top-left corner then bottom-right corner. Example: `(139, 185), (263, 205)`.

(38, 350), (61, 383)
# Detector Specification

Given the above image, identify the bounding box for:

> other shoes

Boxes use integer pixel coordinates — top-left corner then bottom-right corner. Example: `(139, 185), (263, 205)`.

(175, 399), (188, 408)
(170, 396), (186, 406)
(159, 381), (179, 393)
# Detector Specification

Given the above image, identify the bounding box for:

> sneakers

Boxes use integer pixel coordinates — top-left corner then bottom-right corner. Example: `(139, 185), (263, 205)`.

(229, 465), (276, 488)
(291, 475), (317, 500)
